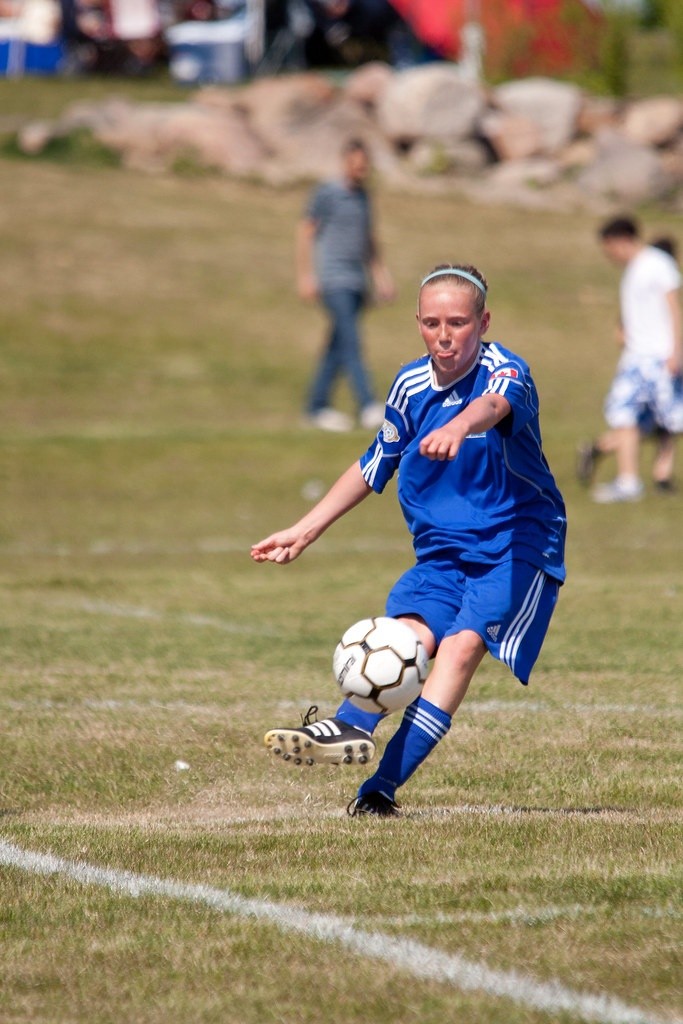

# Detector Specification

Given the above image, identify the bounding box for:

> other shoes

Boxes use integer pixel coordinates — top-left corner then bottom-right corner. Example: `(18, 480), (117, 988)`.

(312, 411), (351, 432)
(576, 444), (602, 487)
(362, 401), (388, 433)
(591, 476), (642, 505)
(653, 480), (671, 493)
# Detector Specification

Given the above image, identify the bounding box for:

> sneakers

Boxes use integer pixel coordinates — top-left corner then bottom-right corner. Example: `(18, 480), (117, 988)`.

(266, 705), (375, 766)
(347, 792), (401, 819)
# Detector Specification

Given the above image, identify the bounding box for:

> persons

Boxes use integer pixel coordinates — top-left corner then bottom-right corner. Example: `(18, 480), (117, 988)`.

(592, 214), (683, 503)
(579, 234), (683, 489)
(295, 140), (397, 432)
(251, 265), (567, 820)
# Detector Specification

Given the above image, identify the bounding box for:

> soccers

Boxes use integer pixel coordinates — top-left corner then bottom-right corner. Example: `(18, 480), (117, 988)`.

(332, 616), (429, 714)
